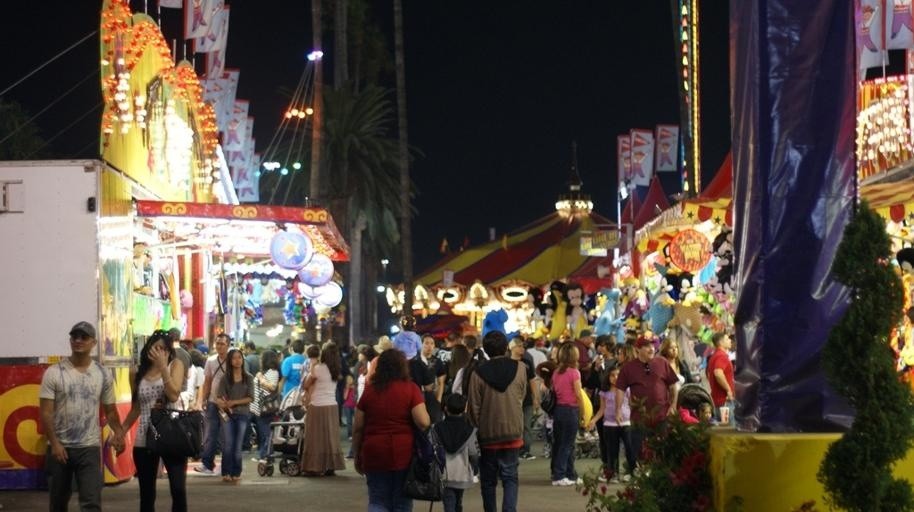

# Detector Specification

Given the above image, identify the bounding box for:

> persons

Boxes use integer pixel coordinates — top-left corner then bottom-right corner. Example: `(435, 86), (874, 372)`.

(170, 328), (347, 481)
(342, 316), (540, 483)
(526, 331), (734, 486)
(351, 349), (431, 512)
(468, 331), (528, 512)
(108, 329), (187, 511)
(39, 320), (126, 512)
(427, 394), (478, 512)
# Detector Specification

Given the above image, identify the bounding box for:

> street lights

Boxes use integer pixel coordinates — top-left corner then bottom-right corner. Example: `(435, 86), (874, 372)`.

(617, 182), (629, 237)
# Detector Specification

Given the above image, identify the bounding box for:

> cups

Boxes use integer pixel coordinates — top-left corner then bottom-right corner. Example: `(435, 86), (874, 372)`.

(719, 407), (729, 424)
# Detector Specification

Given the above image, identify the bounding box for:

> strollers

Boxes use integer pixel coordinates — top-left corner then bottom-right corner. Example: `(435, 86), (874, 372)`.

(676, 383), (715, 421)
(256, 388), (307, 477)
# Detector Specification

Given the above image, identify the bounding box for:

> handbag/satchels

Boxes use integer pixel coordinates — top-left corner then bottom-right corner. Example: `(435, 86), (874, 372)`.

(259, 392), (280, 417)
(542, 390), (556, 413)
(146, 409), (201, 457)
(403, 453), (444, 501)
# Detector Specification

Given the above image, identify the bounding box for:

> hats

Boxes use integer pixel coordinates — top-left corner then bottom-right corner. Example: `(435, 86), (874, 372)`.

(373, 334), (393, 354)
(508, 338), (523, 350)
(70, 322), (95, 338)
(636, 336), (657, 346)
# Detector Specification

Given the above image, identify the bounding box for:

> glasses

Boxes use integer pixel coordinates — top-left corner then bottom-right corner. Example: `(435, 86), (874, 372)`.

(645, 363), (650, 374)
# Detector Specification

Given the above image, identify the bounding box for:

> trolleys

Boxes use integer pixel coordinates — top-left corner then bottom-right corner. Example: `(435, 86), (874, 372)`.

(543, 412), (601, 460)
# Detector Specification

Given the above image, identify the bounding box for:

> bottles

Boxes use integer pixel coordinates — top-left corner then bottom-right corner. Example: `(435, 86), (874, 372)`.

(599, 353), (605, 370)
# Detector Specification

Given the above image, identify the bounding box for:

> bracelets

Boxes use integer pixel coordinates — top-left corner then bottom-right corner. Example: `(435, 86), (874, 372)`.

(163, 376), (171, 384)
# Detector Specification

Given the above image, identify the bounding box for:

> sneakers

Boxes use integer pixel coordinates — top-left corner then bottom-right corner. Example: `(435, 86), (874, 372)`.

(551, 477), (583, 486)
(194, 465), (214, 475)
(598, 471), (620, 484)
(518, 452), (535, 460)
